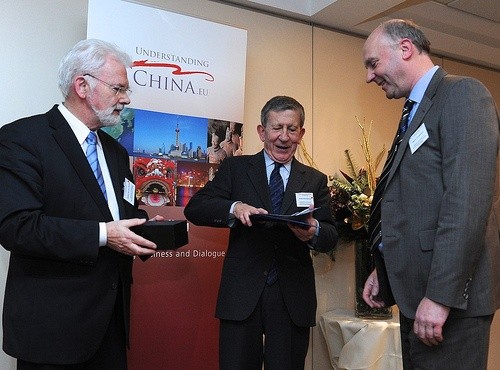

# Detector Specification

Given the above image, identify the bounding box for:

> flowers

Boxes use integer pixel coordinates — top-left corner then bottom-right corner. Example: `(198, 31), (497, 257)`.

(325, 151), (374, 237)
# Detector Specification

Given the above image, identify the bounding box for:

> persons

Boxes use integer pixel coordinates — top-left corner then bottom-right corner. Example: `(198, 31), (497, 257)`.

(184, 95), (338, 369)
(207, 122), (243, 164)
(364, 19), (500, 370)
(0, 38), (167, 370)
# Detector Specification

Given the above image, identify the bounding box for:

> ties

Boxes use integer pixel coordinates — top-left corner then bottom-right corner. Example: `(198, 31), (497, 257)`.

(368, 99), (416, 254)
(85, 132), (107, 202)
(269, 161), (285, 214)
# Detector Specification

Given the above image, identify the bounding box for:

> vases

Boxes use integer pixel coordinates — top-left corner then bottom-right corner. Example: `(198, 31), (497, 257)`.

(354, 234), (392, 321)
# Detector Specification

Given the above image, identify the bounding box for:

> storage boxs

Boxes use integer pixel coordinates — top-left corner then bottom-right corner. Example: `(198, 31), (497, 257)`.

(137, 218), (188, 251)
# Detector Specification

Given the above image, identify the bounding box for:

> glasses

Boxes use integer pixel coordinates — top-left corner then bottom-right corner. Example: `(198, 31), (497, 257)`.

(83, 73), (132, 96)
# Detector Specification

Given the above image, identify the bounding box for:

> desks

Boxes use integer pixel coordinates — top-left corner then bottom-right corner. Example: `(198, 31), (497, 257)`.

(318, 306), (403, 370)
